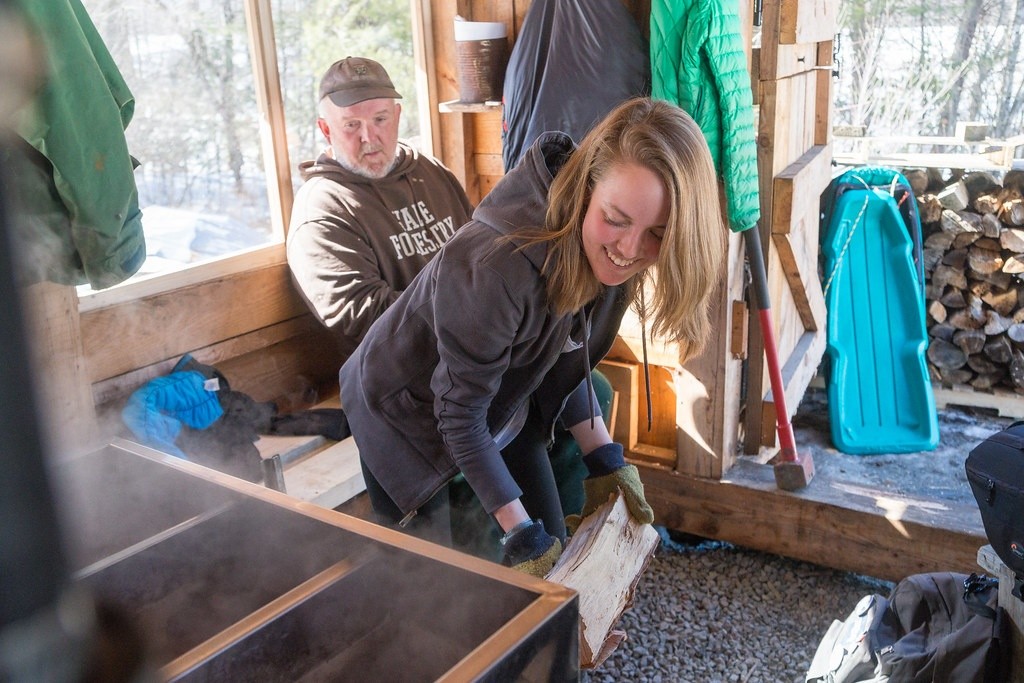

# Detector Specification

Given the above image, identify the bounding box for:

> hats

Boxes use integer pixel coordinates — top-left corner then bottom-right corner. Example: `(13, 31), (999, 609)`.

(317, 56), (402, 107)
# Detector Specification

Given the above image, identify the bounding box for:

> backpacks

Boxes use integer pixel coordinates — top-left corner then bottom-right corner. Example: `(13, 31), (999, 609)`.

(803, 571), (1010, 683)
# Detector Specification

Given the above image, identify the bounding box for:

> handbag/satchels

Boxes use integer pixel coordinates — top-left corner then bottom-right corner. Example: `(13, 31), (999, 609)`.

(965, 420), (1024, 599)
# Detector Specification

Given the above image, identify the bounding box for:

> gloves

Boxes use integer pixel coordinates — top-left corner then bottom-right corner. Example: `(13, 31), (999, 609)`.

(563, 443), (654, 538)
(501, 519), (562, 580)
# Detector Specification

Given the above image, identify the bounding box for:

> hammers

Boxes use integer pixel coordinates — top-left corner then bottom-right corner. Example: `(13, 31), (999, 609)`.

(743, 225), (818, 489)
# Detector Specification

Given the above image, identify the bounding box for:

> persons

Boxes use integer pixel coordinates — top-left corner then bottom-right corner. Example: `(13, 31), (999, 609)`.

(285, 55), (612, 567)
(339, 95), (729, 616)
